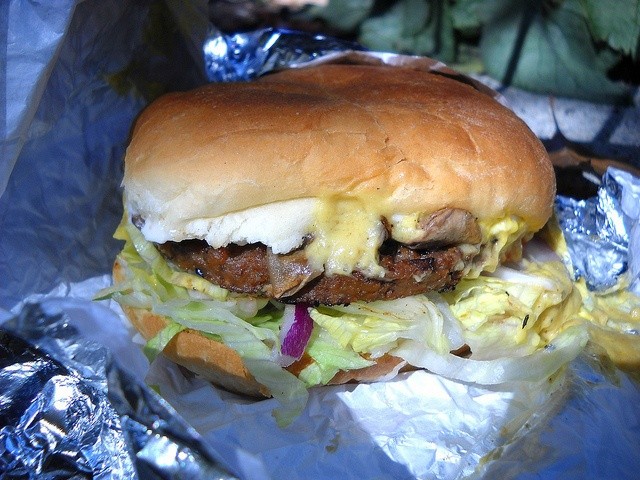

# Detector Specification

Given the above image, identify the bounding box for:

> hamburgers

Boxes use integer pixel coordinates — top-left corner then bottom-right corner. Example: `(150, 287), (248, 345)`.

(91, 63), (639, 430)
